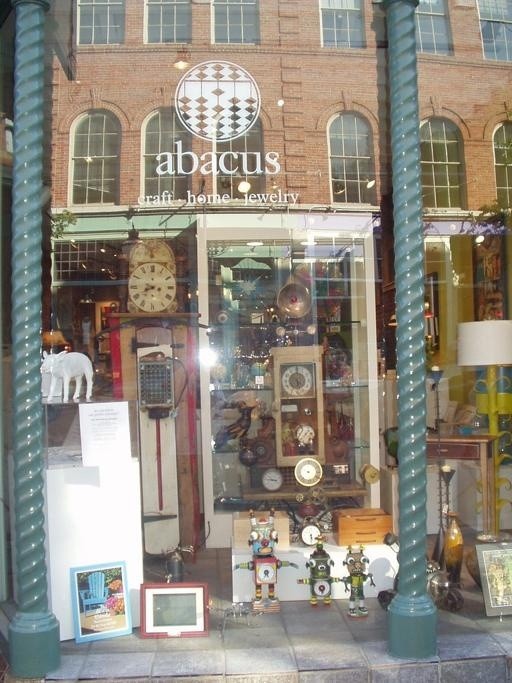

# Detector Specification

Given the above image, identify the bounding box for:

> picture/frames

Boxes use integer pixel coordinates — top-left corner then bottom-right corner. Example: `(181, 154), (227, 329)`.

(473, 542), (511, 619)
(68, 560), (210, 645)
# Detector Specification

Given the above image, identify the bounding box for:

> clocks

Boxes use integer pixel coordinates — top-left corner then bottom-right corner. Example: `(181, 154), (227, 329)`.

(261, 468), (285, 492)
(282, 365), (312, 394)
(129, 262), (178, 315)
(301, 523), (322, 546)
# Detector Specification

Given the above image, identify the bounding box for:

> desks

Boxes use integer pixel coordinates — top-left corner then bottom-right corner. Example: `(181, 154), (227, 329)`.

(427, 434), (500, 543)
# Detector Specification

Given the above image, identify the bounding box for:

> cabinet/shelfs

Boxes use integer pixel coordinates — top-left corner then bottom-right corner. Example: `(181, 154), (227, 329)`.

(189, 212), (383, 548)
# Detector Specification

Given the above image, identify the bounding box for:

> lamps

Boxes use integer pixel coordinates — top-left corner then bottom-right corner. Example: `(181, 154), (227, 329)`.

(455, 319), (512, 542)
